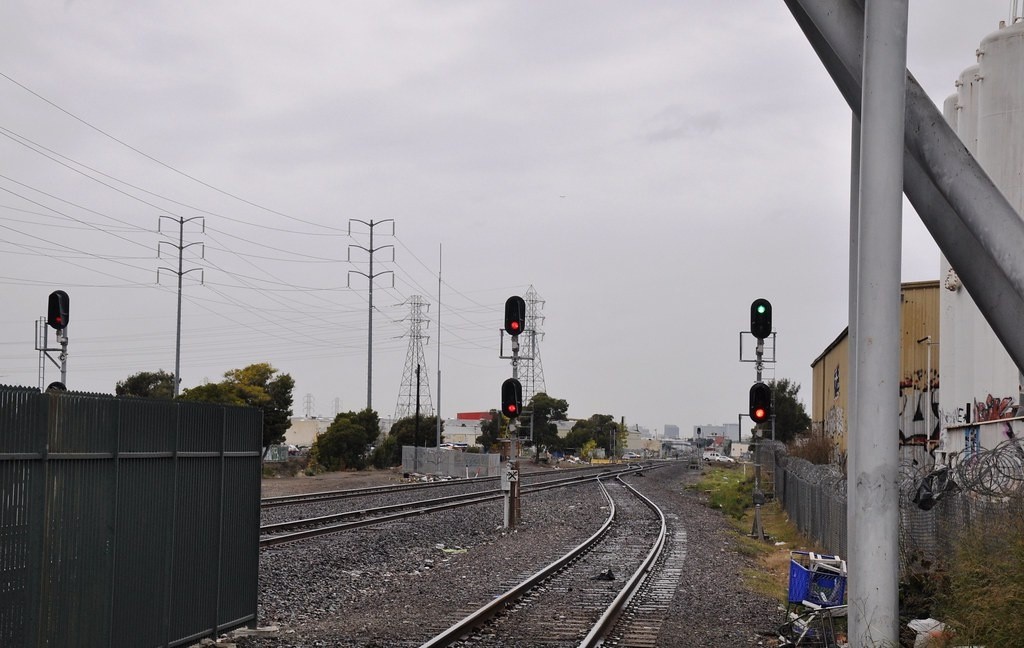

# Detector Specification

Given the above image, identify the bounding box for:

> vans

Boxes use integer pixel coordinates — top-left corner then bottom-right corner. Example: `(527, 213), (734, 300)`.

(702, 451), (720, 461)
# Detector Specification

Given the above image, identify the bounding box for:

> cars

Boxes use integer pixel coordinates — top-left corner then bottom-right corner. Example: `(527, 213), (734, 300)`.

(715, 456), (734, 463)
(628, 451), (641, 458)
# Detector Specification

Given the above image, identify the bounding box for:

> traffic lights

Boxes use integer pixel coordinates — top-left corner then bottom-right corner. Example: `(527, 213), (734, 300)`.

(749, 382), (771, 424)
(751, 298), (773, 339)
(504, 295), (526, 335)
(501, 378), (522, 418)
(47, 290), (70, 330)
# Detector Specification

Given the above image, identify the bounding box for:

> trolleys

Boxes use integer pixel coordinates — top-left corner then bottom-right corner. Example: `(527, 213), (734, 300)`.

(778, 550), (847, 635)
